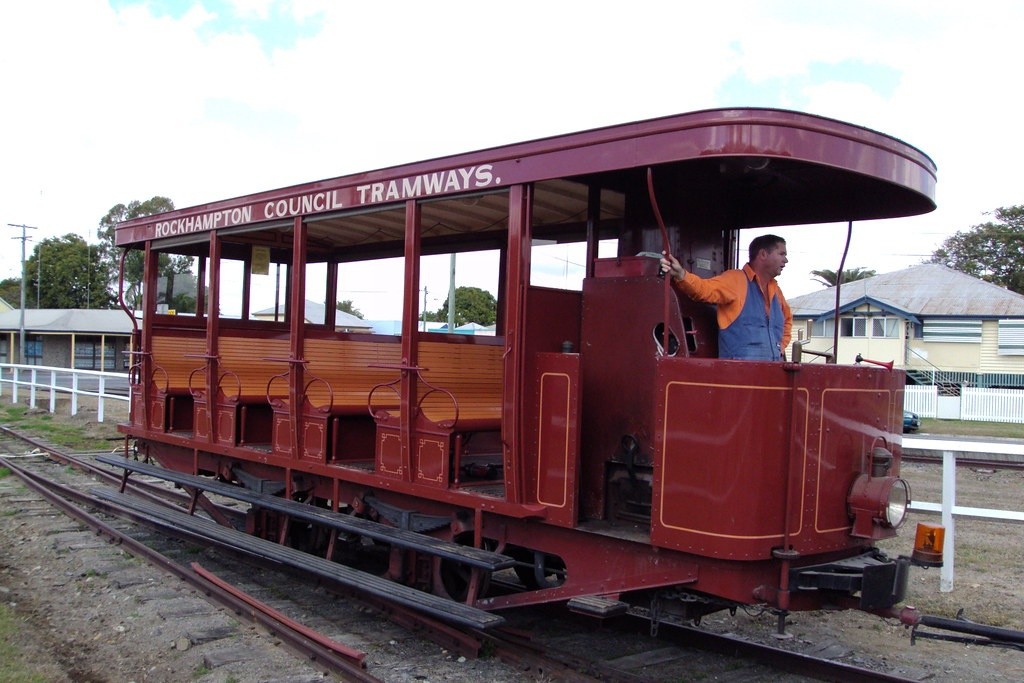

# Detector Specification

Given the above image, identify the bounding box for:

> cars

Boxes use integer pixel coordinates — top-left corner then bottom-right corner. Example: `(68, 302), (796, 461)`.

(903, 410), (921, 433)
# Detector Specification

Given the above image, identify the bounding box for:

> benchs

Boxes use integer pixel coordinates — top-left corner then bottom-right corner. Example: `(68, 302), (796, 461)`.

(151, 327), (206, 394)
(188, 365), (206, 402)
(302, 330), (400, 414)
(265, 370), (289, 412)
(367, 363), (401, 429)
(216, 330), (288, 403)
(415, 332), (502, 432)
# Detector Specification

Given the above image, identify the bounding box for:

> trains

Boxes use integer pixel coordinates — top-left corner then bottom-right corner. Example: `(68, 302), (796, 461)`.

(112, 103), (946, 643)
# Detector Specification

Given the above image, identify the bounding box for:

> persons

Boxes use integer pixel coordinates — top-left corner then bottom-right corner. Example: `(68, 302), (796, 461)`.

(661, 234), (793, 361)
